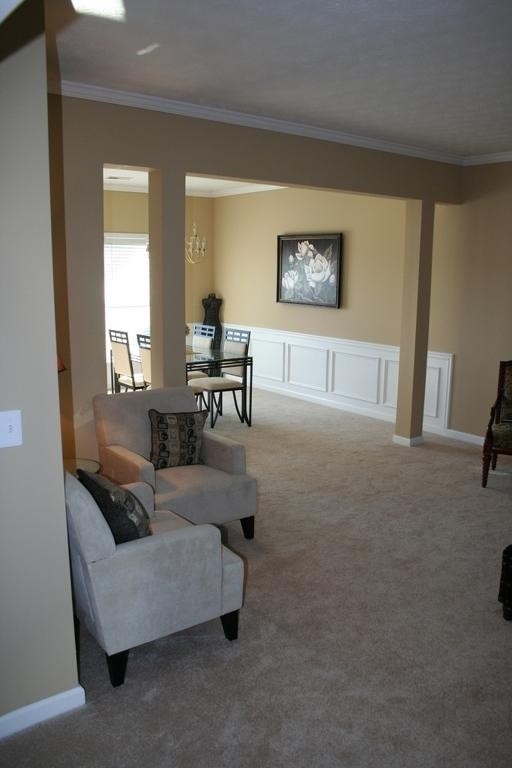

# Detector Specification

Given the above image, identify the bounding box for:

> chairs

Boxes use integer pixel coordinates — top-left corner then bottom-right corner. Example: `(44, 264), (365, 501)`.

(480, 360), (512, 488)
(110, 323), (250, 426)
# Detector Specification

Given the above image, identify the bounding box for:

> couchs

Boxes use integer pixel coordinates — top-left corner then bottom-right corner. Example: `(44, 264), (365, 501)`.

(65, 387), (259, 688)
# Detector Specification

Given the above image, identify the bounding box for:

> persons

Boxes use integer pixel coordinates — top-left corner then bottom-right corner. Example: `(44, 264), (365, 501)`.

(201, 293), (223, 377)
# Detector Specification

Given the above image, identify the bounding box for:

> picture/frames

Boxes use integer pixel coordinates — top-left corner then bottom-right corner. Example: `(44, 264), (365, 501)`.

(276, 234), (343, 310)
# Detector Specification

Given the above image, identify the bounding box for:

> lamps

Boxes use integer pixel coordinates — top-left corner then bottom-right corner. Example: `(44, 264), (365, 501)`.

(185, 221), (209, 265)
